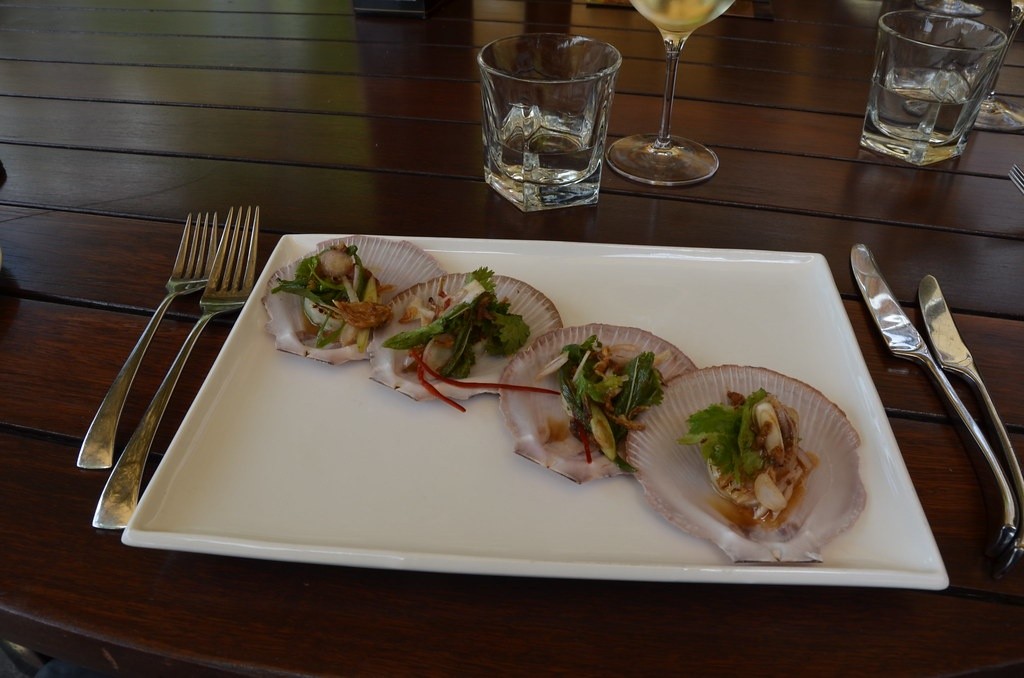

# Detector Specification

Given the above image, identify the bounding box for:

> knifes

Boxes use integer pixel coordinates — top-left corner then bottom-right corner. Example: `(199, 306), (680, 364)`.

(849, 243), (1020, 560)
(918, 273), (1024, 580)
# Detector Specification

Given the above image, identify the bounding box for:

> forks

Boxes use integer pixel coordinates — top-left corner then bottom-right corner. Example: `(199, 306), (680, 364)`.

(77, 211), (218, 469)
(92, 205), (261, 530)
(1008, 163), (1024, 195)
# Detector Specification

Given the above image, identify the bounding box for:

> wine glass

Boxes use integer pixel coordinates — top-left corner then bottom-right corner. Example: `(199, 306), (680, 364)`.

(605, 0), (737, 187)
(903, 0), (1024, 131)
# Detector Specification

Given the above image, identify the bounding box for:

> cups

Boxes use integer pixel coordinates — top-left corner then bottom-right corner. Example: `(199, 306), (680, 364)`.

(859, 10), (1009, 167)
(478, 32), (623, 213)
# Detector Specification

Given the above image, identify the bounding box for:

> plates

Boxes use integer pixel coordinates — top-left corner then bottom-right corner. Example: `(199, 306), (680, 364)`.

(119, 234), (950, 593)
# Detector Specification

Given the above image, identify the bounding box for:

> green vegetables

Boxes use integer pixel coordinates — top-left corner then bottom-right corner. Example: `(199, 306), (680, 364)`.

(267, 243), (776, 486)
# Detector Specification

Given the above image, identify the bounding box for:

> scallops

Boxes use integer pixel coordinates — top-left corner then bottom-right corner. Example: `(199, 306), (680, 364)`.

(260, 235), (448, 365)
(366, 273), (564, 402)
(624, 364), (868, 564)
(498, 324), (698, 485)
